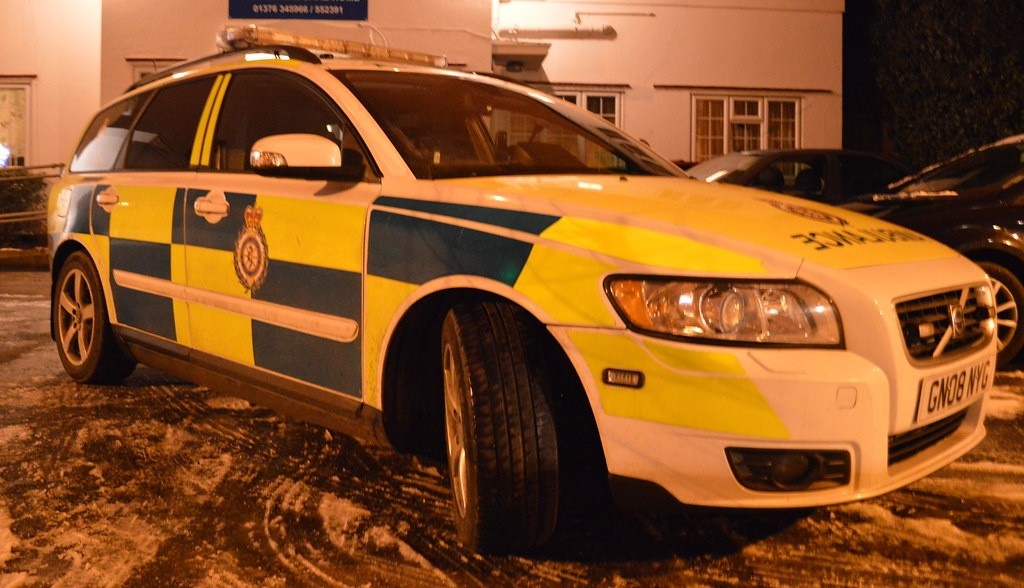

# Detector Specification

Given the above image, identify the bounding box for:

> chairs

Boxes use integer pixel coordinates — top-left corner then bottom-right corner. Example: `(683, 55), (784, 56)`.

(251, 134), (342, 168)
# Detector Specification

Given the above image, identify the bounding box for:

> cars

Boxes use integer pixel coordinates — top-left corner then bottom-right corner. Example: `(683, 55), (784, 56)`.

(42, 24), (1001, 557)
(831, 133), (1024, 370)
(683, 146), (915, 206)
